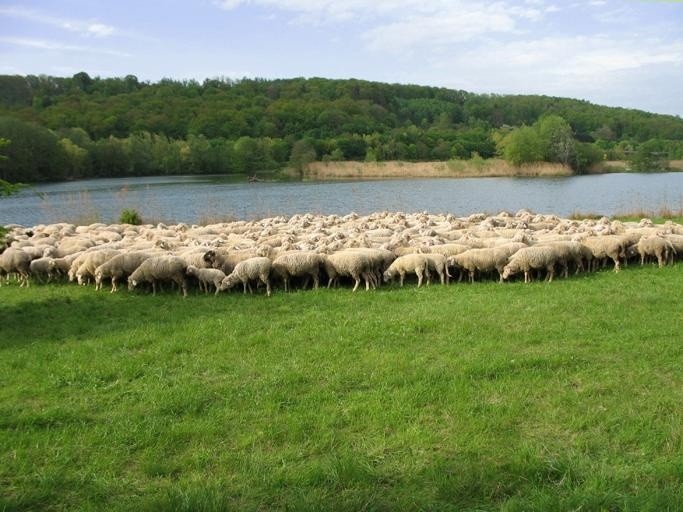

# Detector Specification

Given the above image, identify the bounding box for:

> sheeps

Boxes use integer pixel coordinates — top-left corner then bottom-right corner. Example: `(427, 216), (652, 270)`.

(1, 208), (683, 298)
